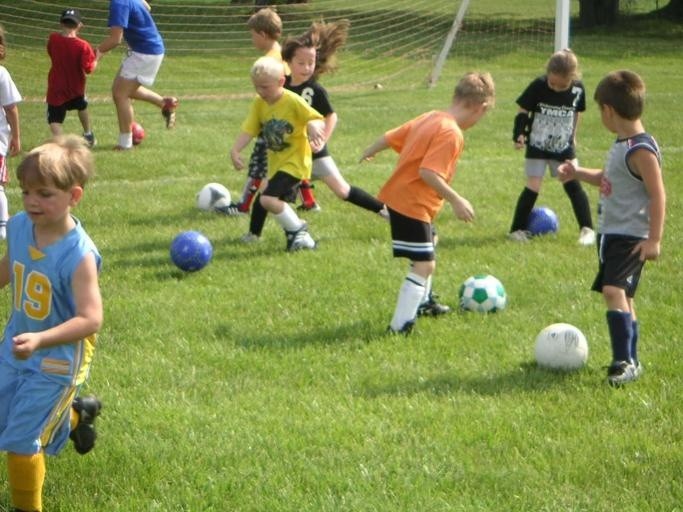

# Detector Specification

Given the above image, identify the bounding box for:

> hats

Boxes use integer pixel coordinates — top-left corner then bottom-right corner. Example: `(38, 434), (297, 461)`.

(61, 9), (81, 25)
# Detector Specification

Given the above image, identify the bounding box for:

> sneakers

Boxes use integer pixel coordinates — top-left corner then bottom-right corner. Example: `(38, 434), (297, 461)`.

(603, 357), (637, 384)
(215, 206), (247, 214)
(70, 396), (101, 454)
(417, 294), (450, 317)
(83, 132), (97, 148)
(578, 227), (595, 245)
(507, 229), (527, 242)
(162, 97), (178, 129)
(284, 202), (320, 251)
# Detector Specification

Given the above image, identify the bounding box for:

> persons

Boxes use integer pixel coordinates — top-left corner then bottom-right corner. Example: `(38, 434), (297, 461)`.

(238, 17), (394, 243)
(355, 74), (497, 339)
(215, 6), (316, 216)
(511, 50), (593, 246)
(230, 56), (325, 252)
(555, 72), (667, 384)
(91, 0), (178, 151)
(0, 22), (24, 246)
(47, 7), (105, 144)
(1, 136), (104, 512)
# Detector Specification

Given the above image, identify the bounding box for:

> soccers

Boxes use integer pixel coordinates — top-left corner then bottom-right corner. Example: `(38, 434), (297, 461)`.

(456, 274), (508, 311)
(131, 121), (147, 147)
(197, 183), (233, 210)
(527, 207), (558, 237)
(535, 322), (589, 369)
(168, 229), (213, 271)
